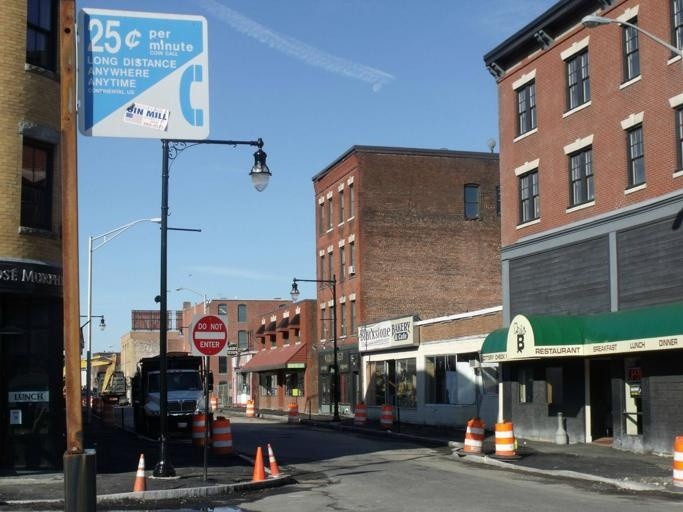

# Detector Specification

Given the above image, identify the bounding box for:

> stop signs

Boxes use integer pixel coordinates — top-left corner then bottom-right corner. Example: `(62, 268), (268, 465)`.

(190, 314), (229, 357)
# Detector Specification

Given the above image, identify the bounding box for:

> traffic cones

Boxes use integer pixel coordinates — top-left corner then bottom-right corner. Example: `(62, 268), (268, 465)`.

(268, 443), (280, 478)
(253, 447), (266, 481)
(133, 453), (146, 492)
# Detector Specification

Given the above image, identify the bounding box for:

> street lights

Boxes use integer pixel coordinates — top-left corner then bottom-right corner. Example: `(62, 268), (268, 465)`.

(153, 137), (273, 477)
(290, 277), (341, 422)
(176, 287), (206, 313)
(80, 217), (163, 429)
(80, 314), (106, 331)
(581, 15), (683, 58)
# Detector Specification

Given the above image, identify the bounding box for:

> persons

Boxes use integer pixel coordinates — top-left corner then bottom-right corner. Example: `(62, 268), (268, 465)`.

(81, 385), (87, 405)
(240, 384), (247, 403)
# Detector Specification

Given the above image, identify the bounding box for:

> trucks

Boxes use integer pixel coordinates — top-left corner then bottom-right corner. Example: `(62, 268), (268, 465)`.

(131, 351), (214, 437)
(95, 369), (126, 406)
